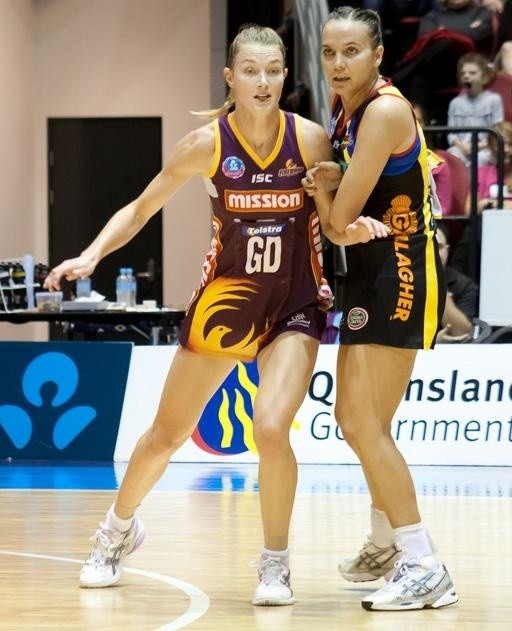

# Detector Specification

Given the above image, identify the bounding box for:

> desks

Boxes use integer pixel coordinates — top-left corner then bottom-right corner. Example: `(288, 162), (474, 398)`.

(0, 308), (187, 341)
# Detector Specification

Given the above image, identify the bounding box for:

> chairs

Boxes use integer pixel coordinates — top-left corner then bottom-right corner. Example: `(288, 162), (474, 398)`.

(416, 72), (512, 216)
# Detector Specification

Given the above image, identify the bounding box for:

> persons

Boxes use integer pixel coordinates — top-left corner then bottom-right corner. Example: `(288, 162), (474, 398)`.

(297, 5), (461, 616)
(444, 52), (504, 173)
(413, 103), (454, 220)
(39, 24), (397, 611)
(416, 0), (495, 62)
(461, 121), (511, 217)
(432, 227), (480, 341)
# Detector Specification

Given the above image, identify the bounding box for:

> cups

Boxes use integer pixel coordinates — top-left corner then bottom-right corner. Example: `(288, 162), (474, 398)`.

(142, 300), (156, 310)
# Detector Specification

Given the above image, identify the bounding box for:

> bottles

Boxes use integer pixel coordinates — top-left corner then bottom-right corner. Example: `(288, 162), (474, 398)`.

(116, 267), (137, 307)
(75, 277), (91, 298)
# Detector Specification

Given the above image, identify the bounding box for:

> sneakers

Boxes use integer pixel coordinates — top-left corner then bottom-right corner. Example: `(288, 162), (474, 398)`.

(361, 559), (459, 612)
(78, 514), (146, 590)
(251, 552), (297, 606)
(338, 540), (401, 582)
